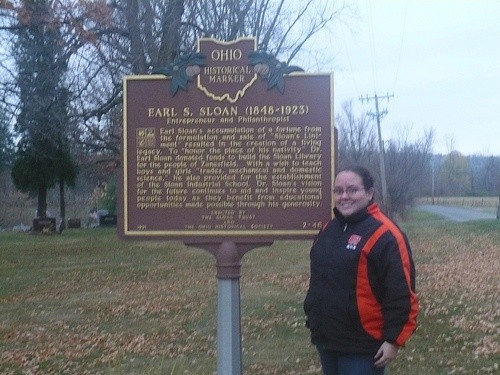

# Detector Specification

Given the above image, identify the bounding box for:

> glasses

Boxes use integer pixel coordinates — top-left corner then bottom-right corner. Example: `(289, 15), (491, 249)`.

(333, 187), (367, 194)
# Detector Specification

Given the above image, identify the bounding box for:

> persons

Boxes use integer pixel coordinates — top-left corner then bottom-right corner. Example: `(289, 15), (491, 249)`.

(87, 207), (100, 229)
(304, 166), (420, 375)
